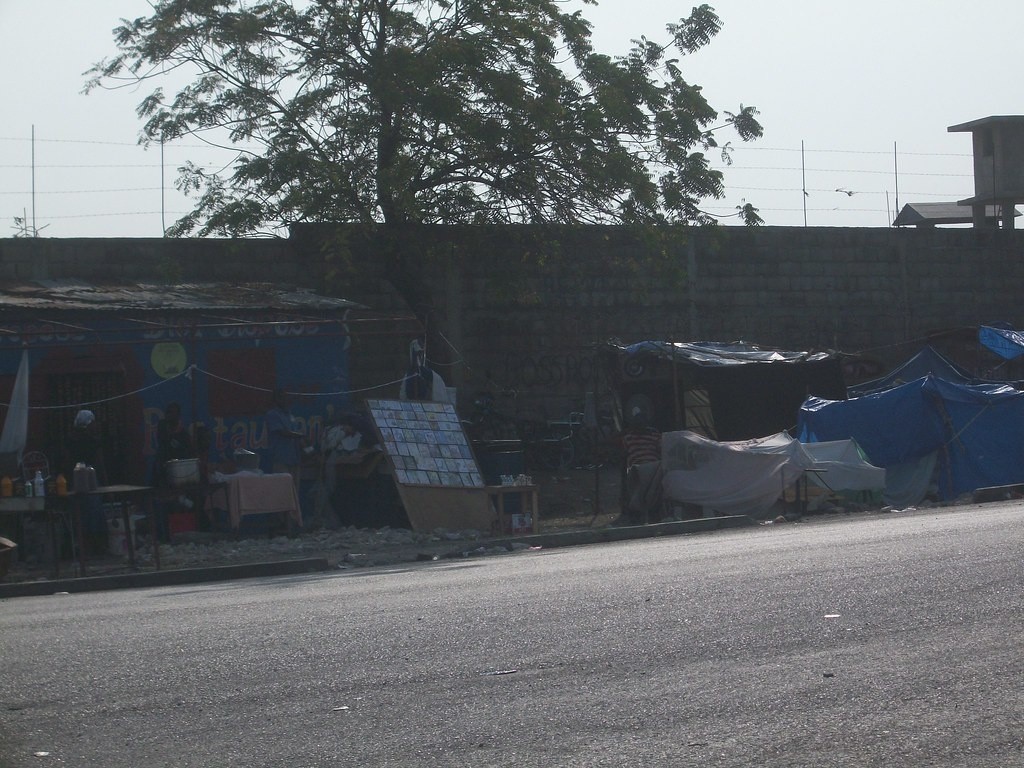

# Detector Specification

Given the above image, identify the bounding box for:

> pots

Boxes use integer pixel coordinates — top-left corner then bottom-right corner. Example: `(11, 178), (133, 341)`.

(234, 453), (258, 468)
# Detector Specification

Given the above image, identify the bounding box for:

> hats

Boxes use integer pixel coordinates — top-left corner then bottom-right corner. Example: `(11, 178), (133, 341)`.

(632, 405), (646, 417)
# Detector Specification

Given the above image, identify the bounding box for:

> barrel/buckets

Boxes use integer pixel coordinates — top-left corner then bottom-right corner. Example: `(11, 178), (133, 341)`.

(512, 512), (530, 534)
(166, 458), (200, 492)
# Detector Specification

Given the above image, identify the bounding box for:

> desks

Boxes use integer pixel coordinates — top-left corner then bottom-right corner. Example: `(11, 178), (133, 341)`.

(487, 485), (540, 537)
(211, 471), (295, 538)
(156, 482), (230, 542)
(85, 485), (161, 573)
(0, 492), (88, 563)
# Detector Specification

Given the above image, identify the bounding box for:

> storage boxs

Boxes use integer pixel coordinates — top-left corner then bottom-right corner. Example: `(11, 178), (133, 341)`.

(0, 536), (18, 577)
(167, 513), (197, 539)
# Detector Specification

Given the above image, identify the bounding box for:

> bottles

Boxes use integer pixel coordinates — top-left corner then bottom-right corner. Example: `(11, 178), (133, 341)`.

(35, 471), (44, 496)
(89, 465), (97, 490)
(57, 474), (66, 495)
(74, 462), (90, 493)
(1, 474), (12, 496)
(25, 480), (32, 497)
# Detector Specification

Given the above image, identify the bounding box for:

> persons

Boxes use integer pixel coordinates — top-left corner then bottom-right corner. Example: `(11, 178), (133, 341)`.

(66, 410), (115, 556)
